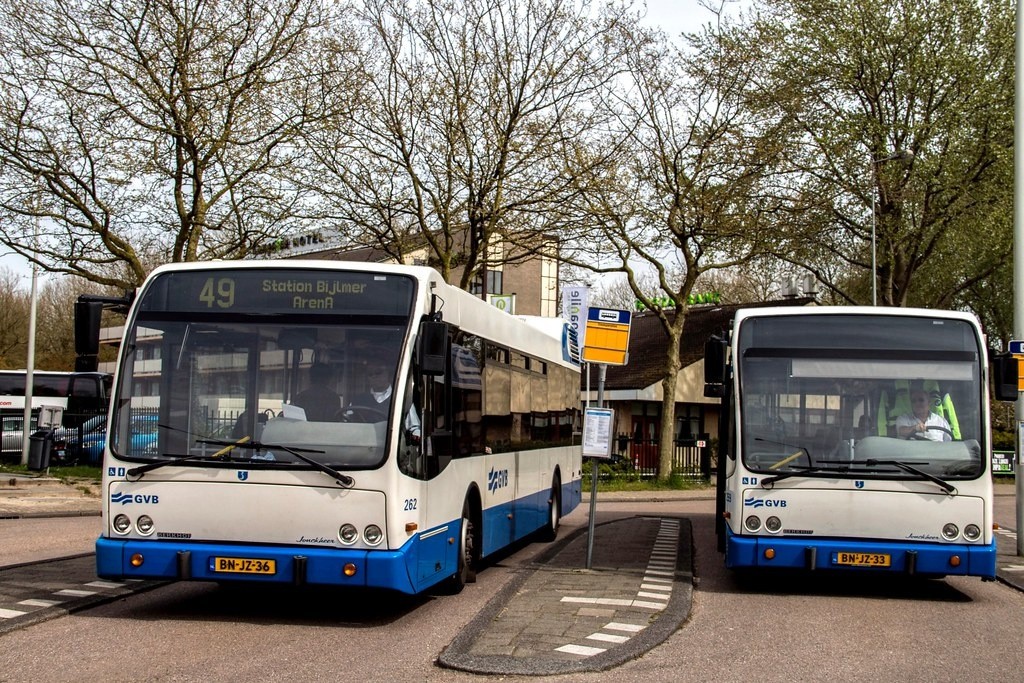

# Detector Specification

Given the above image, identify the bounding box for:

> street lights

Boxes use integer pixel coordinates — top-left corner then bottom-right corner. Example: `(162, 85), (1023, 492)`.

(872, 150), (914, 306)
(20, 175), (133, 466)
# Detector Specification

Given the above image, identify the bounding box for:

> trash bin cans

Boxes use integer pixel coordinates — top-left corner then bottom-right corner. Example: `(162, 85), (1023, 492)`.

(29, 429), (53, 470)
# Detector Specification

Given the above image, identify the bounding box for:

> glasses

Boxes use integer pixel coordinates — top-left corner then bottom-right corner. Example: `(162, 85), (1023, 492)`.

(911, 397), (928, 402)
(367, 367), (389, 377)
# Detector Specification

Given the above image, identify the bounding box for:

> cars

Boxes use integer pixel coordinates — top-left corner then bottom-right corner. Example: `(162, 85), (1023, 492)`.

(65, 415), (148, 463)
(53, 414), (107, 464)
(1, 417), (75, 458)
(90, 416), (159, 462)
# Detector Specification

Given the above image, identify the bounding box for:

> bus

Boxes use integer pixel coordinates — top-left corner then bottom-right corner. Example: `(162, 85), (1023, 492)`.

(703, 304), (1019, 585)
(74, 259), (585, 597)
(1, 369), (113, 429)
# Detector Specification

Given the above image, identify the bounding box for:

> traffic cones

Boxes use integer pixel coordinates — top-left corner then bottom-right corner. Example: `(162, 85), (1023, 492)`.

(635, 454), (641, 471)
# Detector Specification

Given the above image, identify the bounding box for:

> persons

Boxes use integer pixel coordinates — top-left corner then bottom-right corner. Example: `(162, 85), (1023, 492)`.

(895, 388), (952, 441)
(343, 355), (420, 445)
(282, 363), (344, 422)
